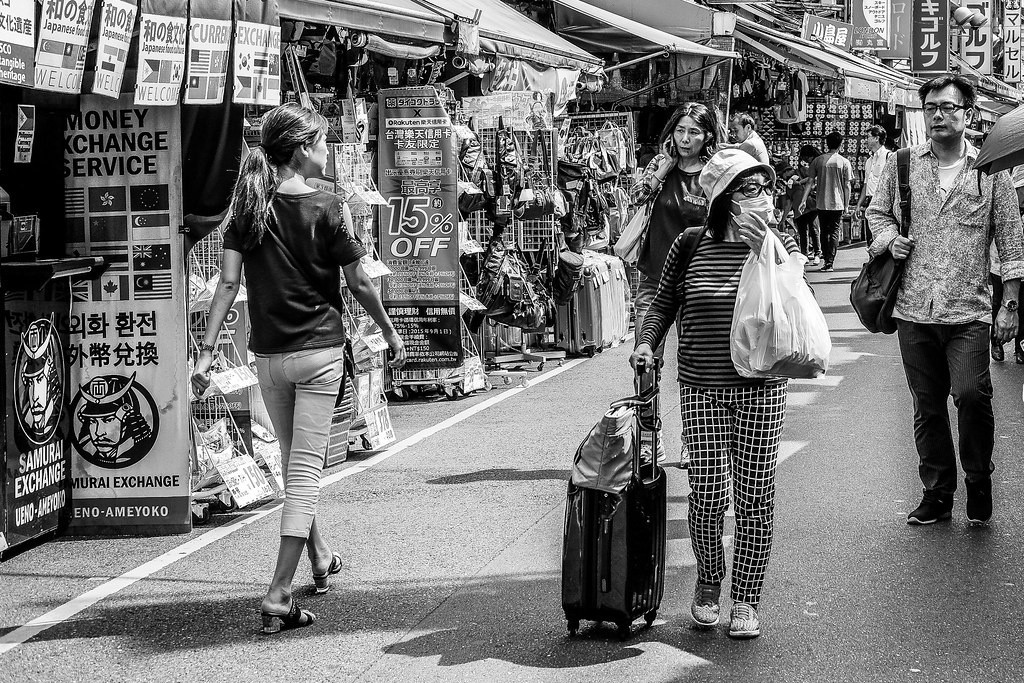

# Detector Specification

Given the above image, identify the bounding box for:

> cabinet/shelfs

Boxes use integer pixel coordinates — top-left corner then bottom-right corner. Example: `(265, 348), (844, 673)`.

(335, 142), (397, 455)
(190, 225), (252, 526)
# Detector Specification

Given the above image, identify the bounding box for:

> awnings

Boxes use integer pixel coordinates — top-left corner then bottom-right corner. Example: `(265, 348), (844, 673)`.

(731, 18), (926, 109)
(278, 0), (605, 75)
(554, 0), (743, 108)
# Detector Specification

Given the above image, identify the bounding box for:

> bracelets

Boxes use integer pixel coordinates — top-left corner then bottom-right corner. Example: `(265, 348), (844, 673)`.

(652, 174), (665, 183)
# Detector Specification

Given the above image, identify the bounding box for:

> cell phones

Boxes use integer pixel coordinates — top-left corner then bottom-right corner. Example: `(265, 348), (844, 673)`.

(670, 135), (676, 158)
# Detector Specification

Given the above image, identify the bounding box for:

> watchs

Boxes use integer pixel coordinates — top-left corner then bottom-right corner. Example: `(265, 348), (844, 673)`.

(1001, 298), (1020, 312)
(199, 341), (214, 350)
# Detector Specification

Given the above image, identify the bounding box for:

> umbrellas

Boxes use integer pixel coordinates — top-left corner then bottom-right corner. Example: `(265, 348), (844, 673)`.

(971, 104), (1024, 177)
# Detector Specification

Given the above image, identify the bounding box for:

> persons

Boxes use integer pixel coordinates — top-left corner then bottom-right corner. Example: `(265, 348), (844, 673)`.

(629, 150), (814, 635)
(717, 112), (769, 165)
(865, 77), (1024, 524)
(192, 102), (406, 633)
(989, 164), (1024, 364)
(798, 132), (855, 271)
(854, 125), (893, 257)
(776, 163), (821, 264)
(630, 102), (719, 467)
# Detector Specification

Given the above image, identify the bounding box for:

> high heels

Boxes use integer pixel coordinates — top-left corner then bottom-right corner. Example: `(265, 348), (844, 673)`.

(261, 597), (316, 633)
(313, 552), (344, 592)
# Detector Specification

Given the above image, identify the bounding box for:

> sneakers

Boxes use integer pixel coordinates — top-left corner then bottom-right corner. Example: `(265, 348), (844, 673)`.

(821, 264), (833, 271)
(689, 578), (721, 625)
(813, 256), (820, 264)
(907, 488), (953, 524)
(729, 603), (760, 636)
(965, 475), (992, 524)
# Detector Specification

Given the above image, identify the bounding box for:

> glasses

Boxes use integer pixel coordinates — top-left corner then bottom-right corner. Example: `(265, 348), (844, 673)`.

(725, 178), (774, 197)
(923, 101), (965, 116)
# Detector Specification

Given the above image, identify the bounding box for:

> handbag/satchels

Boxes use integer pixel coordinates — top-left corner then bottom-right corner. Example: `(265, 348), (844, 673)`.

(613, 184), (662, 263)
(455, 112), (637, 358)
(571, 394), (666, 494)
(730, 229), (832, 379)
(561, 357), (667, 641)
(850, 148), (910, 334)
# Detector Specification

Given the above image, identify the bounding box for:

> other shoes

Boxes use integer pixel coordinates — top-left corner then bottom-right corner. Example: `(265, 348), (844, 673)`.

(991, 344), (1004, 361)
(1016, 353), (1024, 364)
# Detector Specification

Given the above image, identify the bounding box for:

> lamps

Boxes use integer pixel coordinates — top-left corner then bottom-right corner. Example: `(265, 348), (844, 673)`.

(970, 13), (988, 31)
(952, 7), (975, 27)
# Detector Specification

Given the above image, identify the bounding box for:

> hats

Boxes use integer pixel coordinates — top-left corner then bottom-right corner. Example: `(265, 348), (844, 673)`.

(698, 149), (777, 216)
